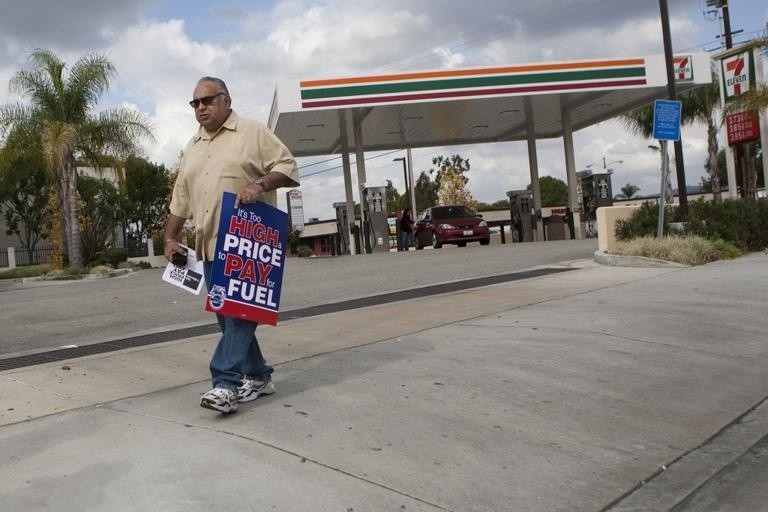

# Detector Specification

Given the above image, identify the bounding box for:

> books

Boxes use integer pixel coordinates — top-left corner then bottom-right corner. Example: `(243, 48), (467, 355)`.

(162, 243), (206, 296)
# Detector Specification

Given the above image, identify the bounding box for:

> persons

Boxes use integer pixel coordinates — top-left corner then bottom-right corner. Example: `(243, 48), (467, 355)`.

(400, 208), (415, 251)
(162, 76), (301, 414)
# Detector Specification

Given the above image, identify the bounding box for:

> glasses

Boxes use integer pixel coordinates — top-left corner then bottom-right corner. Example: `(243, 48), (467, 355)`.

(189, 91), (227, 108)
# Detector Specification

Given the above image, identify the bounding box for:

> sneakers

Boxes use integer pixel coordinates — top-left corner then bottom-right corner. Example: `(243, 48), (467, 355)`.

(199, 387), (239, 414)
(236, 376), (276, 402)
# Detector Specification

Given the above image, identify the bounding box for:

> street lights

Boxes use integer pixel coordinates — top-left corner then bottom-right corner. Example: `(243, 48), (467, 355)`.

(393, 157), (414, 247)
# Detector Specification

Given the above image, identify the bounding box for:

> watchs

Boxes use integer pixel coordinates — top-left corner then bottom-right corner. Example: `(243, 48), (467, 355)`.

(253, 174), (267, 192)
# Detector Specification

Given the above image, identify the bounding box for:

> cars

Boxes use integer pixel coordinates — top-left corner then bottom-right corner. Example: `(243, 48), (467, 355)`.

(411, 205), (490, 250)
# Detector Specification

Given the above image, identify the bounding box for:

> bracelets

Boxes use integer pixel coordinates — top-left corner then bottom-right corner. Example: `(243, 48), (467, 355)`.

(165, 238), (174, 243)
